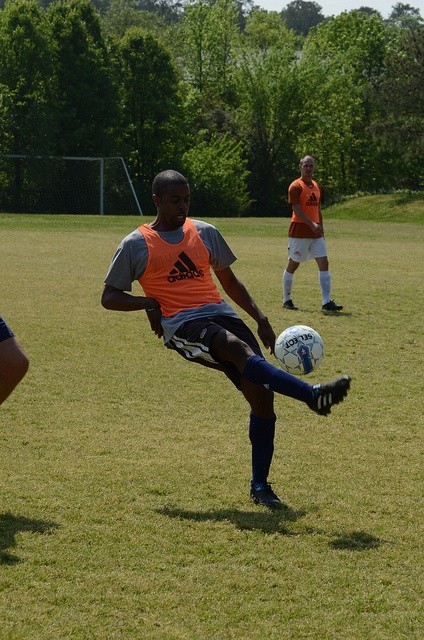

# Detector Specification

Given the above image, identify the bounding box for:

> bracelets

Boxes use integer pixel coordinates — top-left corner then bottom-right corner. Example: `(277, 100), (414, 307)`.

(145, 305), (161, 312)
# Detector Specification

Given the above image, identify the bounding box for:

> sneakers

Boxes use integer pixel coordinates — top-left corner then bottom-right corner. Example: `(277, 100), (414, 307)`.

(283, 301), (297, 310)
(251, 485), (287, 509)
(311, 378), (351, 417)
(321, 302), (343, 310)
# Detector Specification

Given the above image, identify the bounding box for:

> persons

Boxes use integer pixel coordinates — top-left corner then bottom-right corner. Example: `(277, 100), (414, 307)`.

(0, 314), (30, 403)
(100, 169), (351, 513)
(281, 155), (342, 311)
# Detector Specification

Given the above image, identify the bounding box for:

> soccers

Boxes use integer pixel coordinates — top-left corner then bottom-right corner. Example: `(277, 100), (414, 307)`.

(274, 326), (324, 378)
